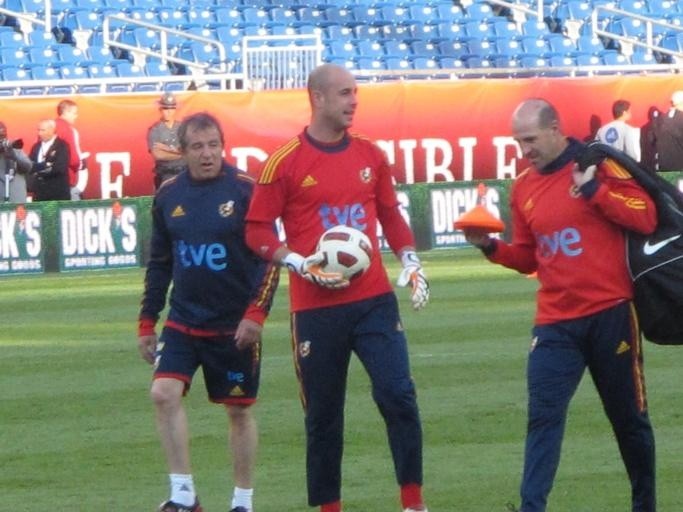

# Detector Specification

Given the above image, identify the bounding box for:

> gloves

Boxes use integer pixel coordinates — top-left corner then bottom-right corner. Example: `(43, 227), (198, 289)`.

(281, 253), (350, 291)
(396, 247), (431, 308)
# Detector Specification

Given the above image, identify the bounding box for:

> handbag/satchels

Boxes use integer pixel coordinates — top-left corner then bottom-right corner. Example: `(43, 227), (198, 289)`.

(627, 219), (683, 346)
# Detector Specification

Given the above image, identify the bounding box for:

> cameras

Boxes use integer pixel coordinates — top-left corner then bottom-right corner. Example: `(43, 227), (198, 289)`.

(2, 139), (24, 150)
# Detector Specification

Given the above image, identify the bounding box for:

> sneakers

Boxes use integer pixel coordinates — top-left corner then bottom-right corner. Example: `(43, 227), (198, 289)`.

(157, 496), (205, 512)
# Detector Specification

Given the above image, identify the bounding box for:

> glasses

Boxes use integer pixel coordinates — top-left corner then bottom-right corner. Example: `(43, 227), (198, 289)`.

(161, 107), (177, 110)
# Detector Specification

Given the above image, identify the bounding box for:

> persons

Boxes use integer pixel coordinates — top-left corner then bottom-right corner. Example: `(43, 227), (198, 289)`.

(465, 98), (658, 512)
(27, 119), (71, 202)
(649, 90), (683, 172)
(244, 64), (431, 512)
(0, 121), (32, 203)
(53, 100), (89, 200)
(137, 112), (281, 512)
(147, 94), (188, 191)
(594, 100), (641, 163)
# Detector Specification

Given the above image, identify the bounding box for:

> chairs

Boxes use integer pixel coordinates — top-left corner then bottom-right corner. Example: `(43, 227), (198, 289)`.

(1, 0), (681, 96)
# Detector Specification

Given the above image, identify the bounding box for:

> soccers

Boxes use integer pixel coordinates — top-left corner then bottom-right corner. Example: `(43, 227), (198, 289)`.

(315, 224), (373, 282)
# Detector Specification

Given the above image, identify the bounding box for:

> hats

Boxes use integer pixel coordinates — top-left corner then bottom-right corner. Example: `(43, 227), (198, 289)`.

(160, 94), (177, 106)
